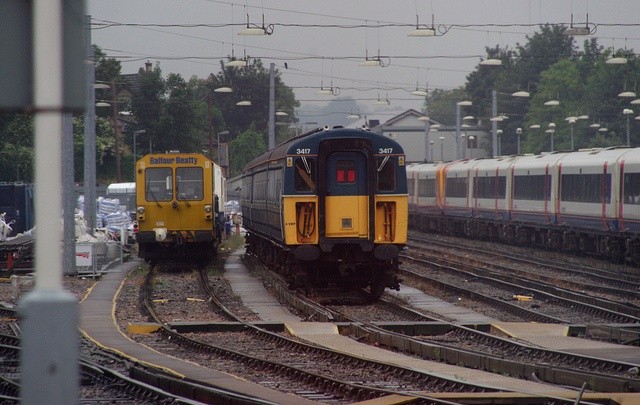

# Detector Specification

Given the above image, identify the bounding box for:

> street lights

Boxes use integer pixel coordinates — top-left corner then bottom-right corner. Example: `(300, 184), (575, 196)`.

(217, 130), (229, 164)
(133, 129), (146, 179)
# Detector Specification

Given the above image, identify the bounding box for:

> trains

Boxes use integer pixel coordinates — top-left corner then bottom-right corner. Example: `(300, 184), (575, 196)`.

(137, 153), (228, 260)
(404, 148), (602, 260)
(241, 126), (409, 300)
